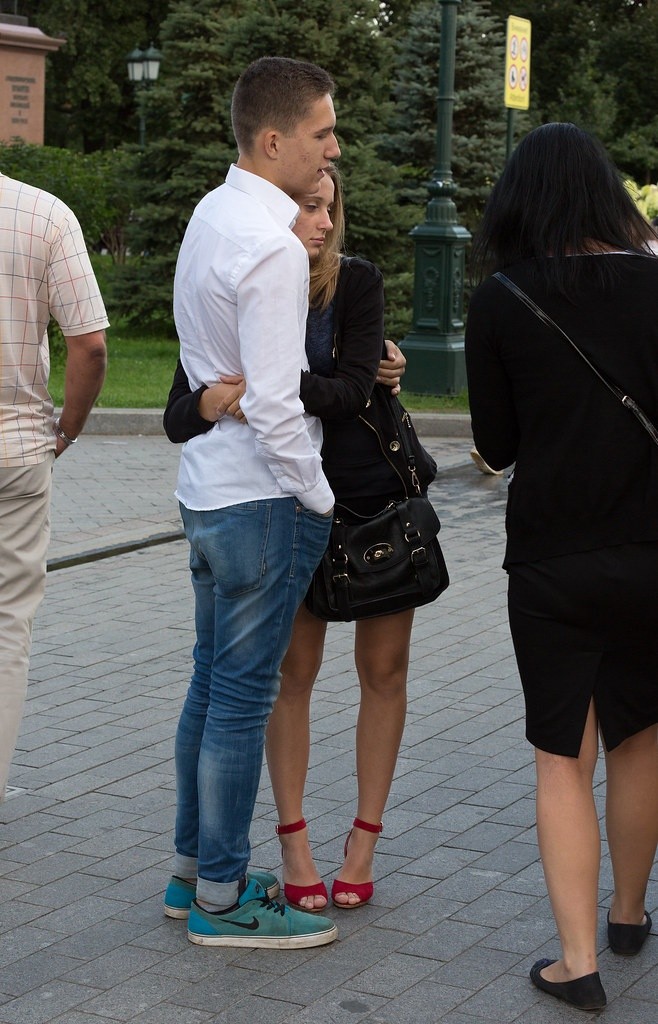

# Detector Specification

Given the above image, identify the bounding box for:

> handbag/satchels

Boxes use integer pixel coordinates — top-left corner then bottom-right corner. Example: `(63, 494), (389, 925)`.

(304, 494), (452, 625)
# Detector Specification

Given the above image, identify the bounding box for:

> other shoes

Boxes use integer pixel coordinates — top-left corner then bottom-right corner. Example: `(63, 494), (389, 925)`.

(529, 907), (652, 1009)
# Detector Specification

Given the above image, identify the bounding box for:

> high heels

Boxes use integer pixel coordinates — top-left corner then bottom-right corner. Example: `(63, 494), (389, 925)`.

(274, 815), (382, 914)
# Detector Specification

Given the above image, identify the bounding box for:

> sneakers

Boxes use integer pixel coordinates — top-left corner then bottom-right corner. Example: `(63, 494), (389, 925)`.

(164, 871), (337, 949)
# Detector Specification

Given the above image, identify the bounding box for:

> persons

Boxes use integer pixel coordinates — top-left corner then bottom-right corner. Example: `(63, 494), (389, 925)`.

(1, 167), (114, 865)
(162, 154), (449, 917)
(158, 59), (341, 954)
(457, 119), (658, 1014)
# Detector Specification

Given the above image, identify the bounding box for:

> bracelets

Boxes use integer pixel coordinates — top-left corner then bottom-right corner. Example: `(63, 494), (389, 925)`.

(54, 417), (78, 445)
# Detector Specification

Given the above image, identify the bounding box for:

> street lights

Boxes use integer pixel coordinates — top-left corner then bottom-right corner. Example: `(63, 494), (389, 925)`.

(127, 40), (162, 147)
